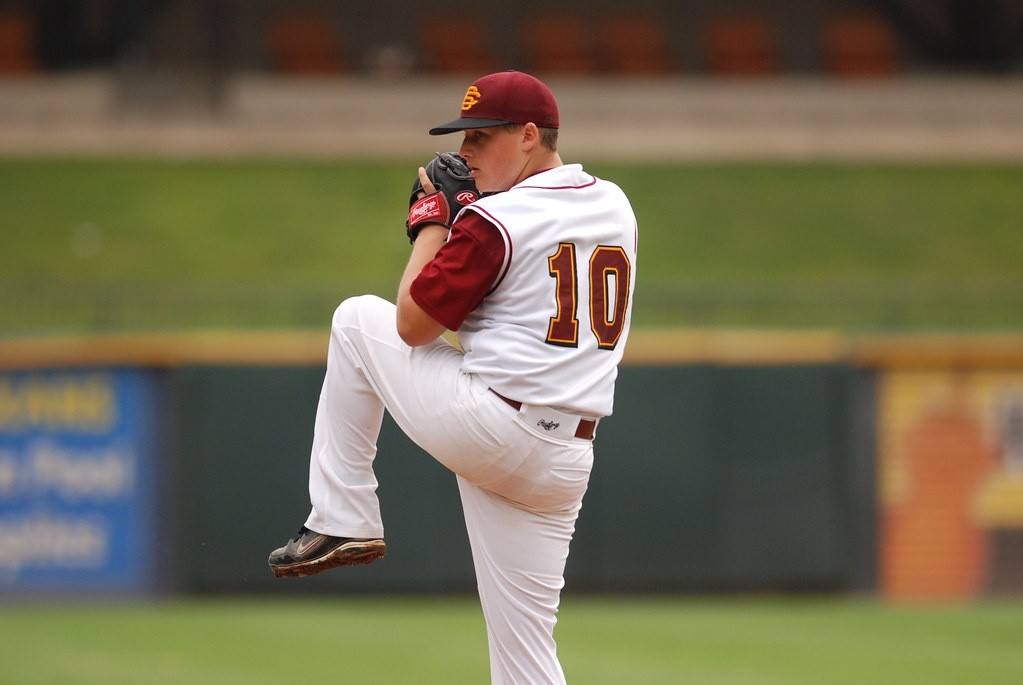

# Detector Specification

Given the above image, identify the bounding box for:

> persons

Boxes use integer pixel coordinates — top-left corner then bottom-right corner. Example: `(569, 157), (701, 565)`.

(267, 67), (638, 685)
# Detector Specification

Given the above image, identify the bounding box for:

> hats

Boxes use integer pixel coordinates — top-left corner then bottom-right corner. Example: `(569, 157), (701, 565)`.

(428, 70), (559, 136)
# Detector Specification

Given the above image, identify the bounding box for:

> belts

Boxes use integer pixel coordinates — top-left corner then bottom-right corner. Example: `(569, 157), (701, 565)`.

(487, 387), (595, 441)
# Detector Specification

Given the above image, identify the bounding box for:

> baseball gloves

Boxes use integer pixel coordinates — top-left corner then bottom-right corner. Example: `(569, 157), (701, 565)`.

(406, 152), (482, 240)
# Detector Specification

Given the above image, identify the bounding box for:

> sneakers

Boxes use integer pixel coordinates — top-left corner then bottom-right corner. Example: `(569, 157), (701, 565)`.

(268, 528), (386, 577)
